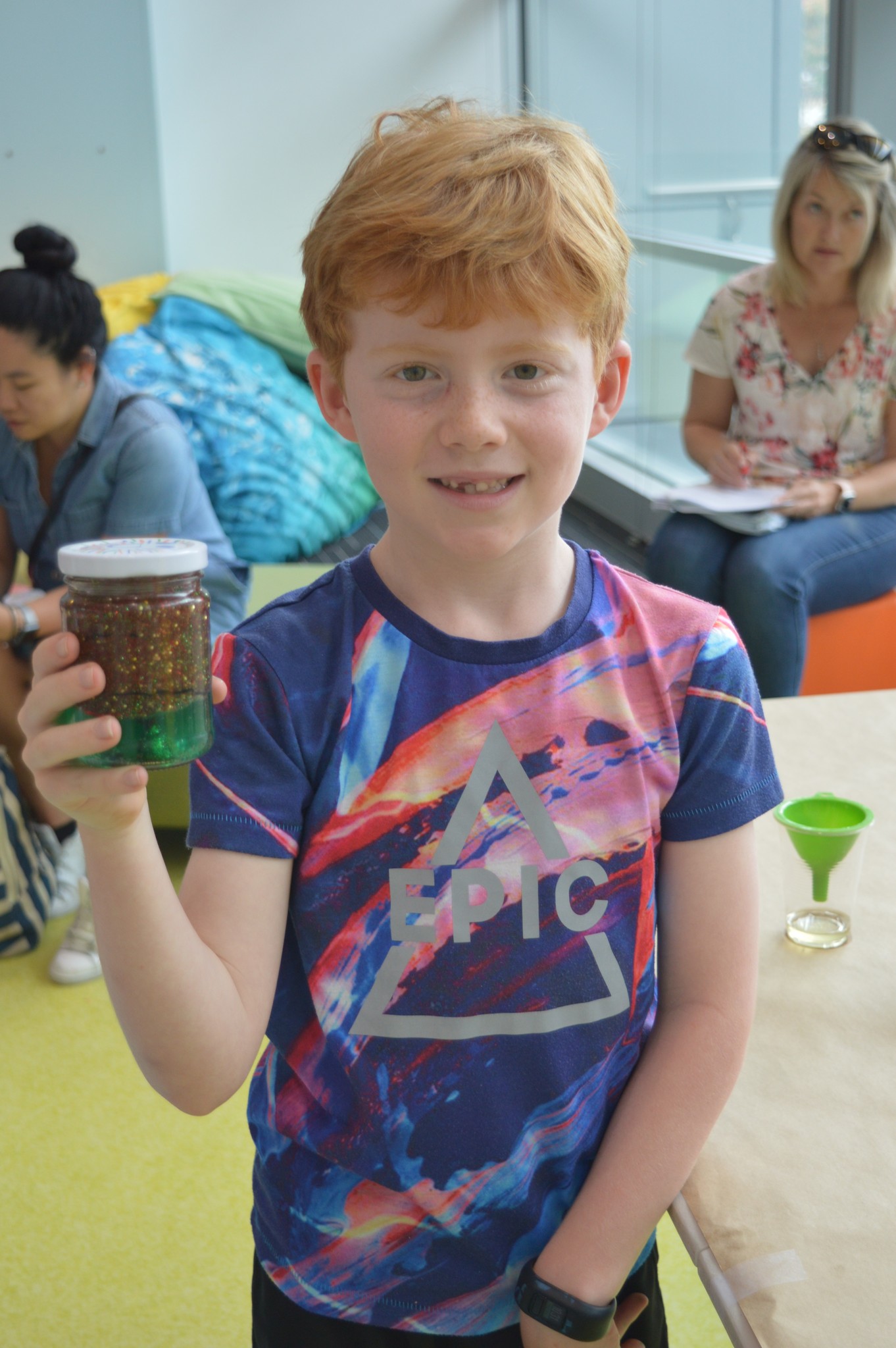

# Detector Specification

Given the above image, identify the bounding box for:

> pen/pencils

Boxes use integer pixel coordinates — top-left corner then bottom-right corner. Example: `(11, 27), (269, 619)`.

(739, 440), (749, 474)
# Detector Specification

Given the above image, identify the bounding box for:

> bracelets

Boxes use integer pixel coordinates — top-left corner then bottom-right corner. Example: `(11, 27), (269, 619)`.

(2, 598), (40, 637)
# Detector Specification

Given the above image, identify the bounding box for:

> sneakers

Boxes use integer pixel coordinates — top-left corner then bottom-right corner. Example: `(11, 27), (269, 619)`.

(48, 878), (103, 984)
(46, 823), (89, 919)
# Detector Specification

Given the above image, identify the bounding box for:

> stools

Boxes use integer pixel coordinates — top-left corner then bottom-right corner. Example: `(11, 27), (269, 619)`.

(145, 561), (335, 828)
(799, 587), (896, 694)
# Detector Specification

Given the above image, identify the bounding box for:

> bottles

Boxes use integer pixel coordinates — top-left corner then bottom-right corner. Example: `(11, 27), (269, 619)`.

(56, 536), (217, 772)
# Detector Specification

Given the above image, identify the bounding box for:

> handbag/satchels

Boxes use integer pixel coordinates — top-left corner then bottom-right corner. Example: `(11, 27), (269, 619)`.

(0, 747), (65, 956)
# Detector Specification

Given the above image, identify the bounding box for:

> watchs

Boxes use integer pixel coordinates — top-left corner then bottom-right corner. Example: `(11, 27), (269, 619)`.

(511, 1259), (619, 1343)
(830, 477), (854, 516)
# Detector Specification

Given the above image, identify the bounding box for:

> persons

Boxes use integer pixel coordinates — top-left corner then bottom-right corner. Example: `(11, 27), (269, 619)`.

(0, 227), (254, 986)
(23, 85), (790, 1348)
(644, 114), (896, 698)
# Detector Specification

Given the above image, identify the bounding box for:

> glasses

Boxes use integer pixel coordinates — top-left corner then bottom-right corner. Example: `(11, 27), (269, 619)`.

(811, 123), (893, 164)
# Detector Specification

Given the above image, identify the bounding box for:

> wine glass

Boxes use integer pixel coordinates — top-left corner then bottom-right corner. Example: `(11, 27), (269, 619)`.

(772, 793), (876, 950)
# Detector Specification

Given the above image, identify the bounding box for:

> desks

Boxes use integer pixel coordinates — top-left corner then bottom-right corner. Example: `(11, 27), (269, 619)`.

(665, 689), (896, 1347)
